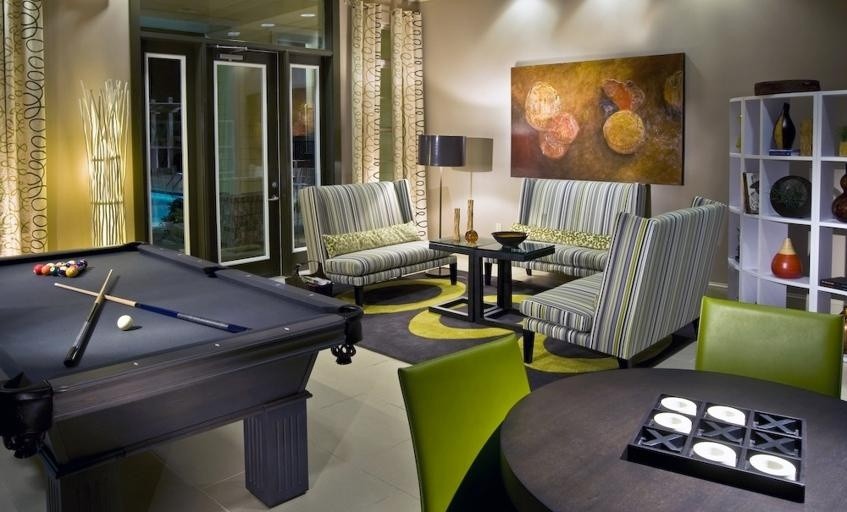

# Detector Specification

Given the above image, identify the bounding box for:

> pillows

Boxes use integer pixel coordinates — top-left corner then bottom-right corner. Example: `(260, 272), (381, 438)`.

(320, 220), (424, 259)
(509, 222), (613, 250)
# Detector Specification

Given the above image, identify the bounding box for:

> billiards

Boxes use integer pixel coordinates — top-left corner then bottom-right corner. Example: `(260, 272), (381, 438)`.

(33, 258), (87, 278)
(117, 315), (133, 330)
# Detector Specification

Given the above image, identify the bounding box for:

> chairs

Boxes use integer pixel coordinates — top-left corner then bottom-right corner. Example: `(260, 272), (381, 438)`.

(220, 190), (261, 248)
(395, 334), (535, 511)
(695, 294), (845, 402)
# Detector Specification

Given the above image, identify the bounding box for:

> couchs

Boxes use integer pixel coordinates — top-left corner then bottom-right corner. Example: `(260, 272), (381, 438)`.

(297, 179), (458, 306)
(518, 192), (728, 365)
(482, 178), (649, 287)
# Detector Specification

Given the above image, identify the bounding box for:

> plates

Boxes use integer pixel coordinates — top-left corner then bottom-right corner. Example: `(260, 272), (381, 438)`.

(769, 176), (811, 219)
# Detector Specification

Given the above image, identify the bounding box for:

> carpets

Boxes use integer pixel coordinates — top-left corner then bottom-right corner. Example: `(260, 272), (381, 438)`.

(327, 266), (699, 392)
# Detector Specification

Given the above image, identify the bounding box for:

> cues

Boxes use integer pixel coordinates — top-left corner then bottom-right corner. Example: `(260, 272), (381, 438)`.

(75, 78), (130, 249)
(63, 268), (114, 367)
(54, 282), (249, 332)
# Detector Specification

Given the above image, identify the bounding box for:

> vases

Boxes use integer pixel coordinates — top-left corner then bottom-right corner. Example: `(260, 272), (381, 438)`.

(772, 100), (798, 152)
(771, 234), (805, 279)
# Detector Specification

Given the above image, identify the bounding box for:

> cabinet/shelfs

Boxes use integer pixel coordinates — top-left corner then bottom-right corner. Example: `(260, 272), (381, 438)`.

(725, 89), (846, 374)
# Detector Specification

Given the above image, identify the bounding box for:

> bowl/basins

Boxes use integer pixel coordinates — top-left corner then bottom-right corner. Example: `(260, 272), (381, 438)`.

(490, 230), (529, 249)
(753, 79), (820, 96)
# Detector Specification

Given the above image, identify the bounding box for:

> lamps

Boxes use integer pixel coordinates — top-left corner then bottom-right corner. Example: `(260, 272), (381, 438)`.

(416, 133), (467, 277)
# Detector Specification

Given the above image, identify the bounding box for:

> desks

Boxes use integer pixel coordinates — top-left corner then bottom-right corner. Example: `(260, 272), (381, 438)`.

(496, 368), (845, 510)
(427, 235), (556, 334)
(0, 240), (366, 511)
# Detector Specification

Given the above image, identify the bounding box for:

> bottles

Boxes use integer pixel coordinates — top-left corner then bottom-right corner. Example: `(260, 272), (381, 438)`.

(771, 102), (795, 150)
(830, 165), (847, 223)
(770, 237), (802, 279)
(838, 307), (847, 353)
